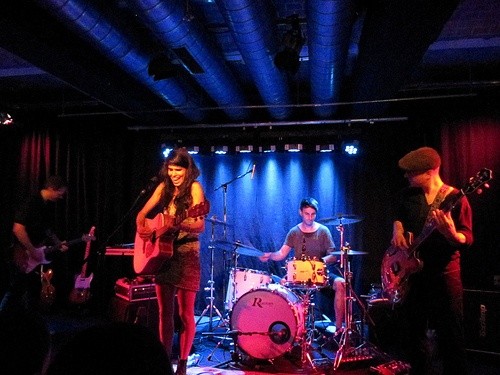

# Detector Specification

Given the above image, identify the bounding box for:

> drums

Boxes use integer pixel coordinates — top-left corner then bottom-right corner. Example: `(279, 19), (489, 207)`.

(224, 266), (274, 315)
(228, 283), (306, 360)
(284, 254), (329, 290)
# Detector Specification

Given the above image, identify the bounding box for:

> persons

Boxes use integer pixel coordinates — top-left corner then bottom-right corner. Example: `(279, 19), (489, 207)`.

(12, 170), (69, 300)
(256, 197), (346, 330)
(390, 147), (474, 375)
(136, 147), (206, 375)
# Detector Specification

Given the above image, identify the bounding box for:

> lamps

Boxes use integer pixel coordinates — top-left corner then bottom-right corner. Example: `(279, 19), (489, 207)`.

(276, 29), (306, 75)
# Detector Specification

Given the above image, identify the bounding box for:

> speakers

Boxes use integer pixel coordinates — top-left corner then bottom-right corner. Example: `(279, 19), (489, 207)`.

(462, 289), (500, 356)
(112, 296), (181, 334)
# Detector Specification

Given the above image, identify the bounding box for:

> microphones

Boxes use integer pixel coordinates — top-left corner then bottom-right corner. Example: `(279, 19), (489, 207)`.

(251, 163), (256, 180)
(141, 177), (157, 195)
(278, 328), (287, 338)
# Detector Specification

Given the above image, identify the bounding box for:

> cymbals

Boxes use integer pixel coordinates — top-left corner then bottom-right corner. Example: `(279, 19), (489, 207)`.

(208, 239), (267, 256)
(313, 213), (370, 229)
(205, 217), (232, 228)
(328, 249), (368, 255)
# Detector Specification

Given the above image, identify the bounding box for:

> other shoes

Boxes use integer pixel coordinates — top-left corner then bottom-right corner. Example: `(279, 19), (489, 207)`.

(174, 358), (187, 375)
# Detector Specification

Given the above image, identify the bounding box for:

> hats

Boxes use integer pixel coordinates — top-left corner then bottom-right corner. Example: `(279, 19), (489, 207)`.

(399, 147), (440, 171)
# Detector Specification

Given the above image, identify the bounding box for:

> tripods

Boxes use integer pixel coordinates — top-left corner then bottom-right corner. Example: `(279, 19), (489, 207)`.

(202, 330), (279, 371)
(317, 252), (390, 375)
(195, 221), (229, 341)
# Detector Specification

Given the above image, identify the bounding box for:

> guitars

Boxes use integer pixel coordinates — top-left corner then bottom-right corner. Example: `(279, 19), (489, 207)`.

(132, 199), (210, 275)
(22, 233), (94, 275)
(380, 167), (493, 304)
(40, 263), (55, 306)
(67, 225), (98, 305)
